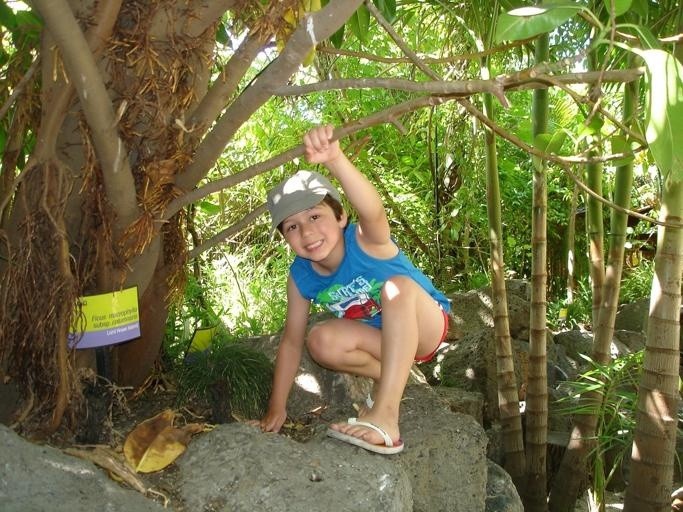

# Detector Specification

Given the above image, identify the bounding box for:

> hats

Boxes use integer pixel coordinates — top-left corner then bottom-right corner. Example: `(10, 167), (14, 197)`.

(266, 170), (338, 234)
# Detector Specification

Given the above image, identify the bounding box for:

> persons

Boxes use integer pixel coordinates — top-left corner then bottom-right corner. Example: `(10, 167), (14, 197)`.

(241, 123), (453, 458)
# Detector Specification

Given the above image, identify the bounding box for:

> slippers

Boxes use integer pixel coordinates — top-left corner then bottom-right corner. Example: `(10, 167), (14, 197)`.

(325, 416), (406, 456)
